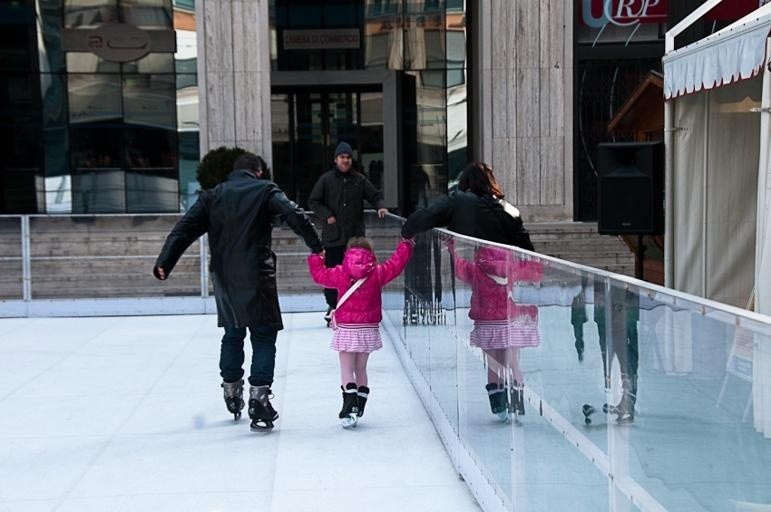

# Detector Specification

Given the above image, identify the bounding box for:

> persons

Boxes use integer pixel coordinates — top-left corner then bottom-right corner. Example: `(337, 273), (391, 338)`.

(307, 236), (418, 428)
(400, 162), (534, 250)
(154, 152), (326, 431)
(579, 269), (640, 416)
(446, 240), (545, 424)
(308, 141), (388, 325)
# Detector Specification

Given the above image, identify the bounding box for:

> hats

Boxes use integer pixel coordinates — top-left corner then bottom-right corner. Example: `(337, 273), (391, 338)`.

(335, 142), (353, 158)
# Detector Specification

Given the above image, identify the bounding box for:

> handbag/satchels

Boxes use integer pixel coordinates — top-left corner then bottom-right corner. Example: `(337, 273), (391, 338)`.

(329, 310), (337, 331)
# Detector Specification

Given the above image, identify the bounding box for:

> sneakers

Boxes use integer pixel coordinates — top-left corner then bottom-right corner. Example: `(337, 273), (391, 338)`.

(583, 404), (596, 417)
(603, 373), (638, 415)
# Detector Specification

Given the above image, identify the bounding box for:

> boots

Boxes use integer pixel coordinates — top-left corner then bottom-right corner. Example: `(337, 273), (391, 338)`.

(339, 383), (368, 418)
(485, 382), (525, 415)
(224, 380), (244, 414)
(249, 386), (278, 421)
